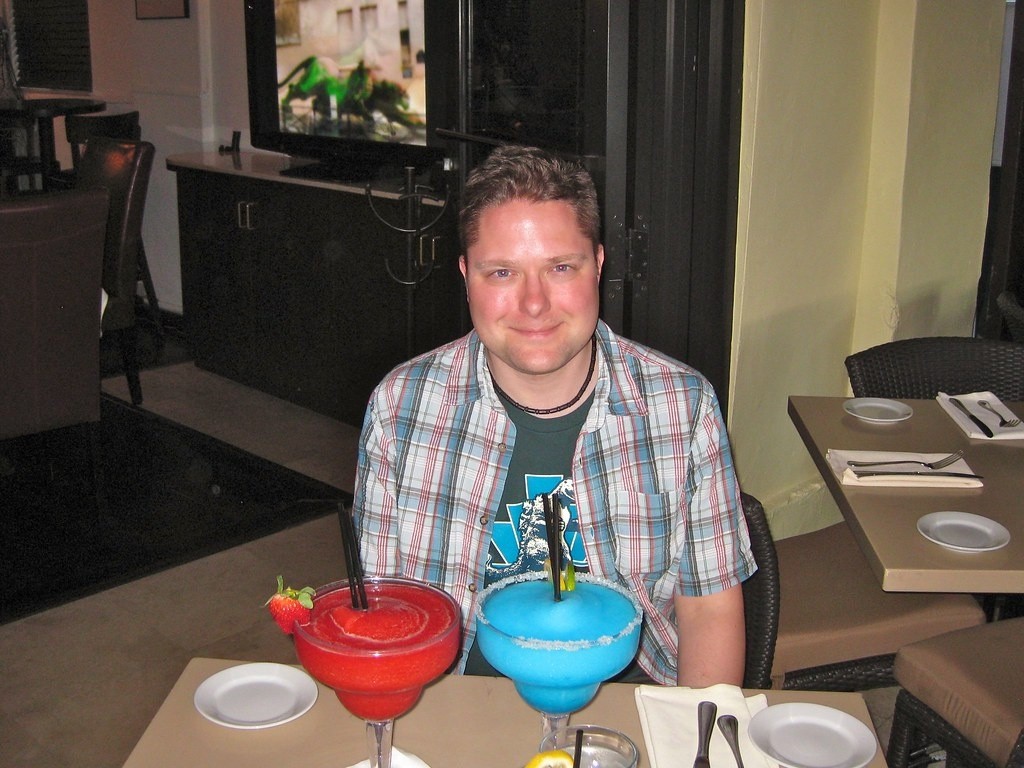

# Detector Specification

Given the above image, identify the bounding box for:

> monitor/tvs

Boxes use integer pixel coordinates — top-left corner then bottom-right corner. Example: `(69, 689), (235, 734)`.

(243, 0), (448, 183)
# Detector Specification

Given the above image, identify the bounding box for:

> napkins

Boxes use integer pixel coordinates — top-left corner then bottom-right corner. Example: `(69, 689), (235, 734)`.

(347, 745), (431, 768)
(633, 684), (779, 768)
(936, 390), (1024, 440)
(826, 448), (984, 488)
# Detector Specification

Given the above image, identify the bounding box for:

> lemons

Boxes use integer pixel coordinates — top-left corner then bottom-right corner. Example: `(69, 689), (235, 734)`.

(542, 558), (576, 591)
(523, 749), (574, 768)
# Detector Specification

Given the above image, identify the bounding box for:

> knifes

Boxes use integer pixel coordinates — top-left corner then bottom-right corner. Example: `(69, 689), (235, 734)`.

(949, 398), (993, 438)
(693, 701), (717, 768)
(852, 471), (985, 479)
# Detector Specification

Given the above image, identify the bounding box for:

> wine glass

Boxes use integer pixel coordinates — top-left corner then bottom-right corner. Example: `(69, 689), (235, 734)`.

(476, 571), (643, 737)
(294, 576), (461, 768)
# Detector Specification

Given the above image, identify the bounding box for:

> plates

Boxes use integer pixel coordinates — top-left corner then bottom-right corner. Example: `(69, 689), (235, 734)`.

(194, 662), (318, 729)
(748, 703), (877, 768)
(843, 397), (913, 424)
(917, 511), (1011, 553)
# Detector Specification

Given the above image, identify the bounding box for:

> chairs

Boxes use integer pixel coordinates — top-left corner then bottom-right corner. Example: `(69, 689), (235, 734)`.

(0, 136), (155, 527)
(734, 336), (1024, 768)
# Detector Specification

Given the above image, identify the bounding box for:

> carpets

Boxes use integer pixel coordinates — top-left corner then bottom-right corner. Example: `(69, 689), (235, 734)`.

(0, 389), (354, 627)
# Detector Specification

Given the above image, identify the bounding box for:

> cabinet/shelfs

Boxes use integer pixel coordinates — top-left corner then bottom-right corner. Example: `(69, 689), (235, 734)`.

(175, 171), (443, 430)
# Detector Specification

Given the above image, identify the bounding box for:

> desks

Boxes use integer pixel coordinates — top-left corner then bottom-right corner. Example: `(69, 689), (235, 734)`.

(117, 658), (889, 768)
(789, 395), (1024, 623)
(0, 98), (106, 195)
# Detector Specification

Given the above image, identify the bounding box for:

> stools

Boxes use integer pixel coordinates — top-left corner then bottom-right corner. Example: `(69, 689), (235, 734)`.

(0, 108), (166, 343)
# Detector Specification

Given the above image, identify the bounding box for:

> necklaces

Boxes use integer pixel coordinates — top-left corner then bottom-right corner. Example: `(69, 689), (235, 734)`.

(486, 334), (598, 415)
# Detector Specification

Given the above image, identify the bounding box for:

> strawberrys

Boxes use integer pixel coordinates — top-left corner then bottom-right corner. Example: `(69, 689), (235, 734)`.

(262, 574), (316, 635)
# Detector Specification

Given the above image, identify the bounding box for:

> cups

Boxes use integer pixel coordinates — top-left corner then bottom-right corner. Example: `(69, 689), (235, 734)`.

(539, 725), (638, 768)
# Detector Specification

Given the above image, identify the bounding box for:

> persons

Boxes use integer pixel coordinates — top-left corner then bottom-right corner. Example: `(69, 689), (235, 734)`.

(351, 145), (758, 694)
(346, 60), (372, 95)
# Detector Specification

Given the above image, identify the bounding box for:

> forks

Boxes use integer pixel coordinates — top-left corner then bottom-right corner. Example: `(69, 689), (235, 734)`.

(977, 400), (1021, 427)
(847, 450), (965, 469)
